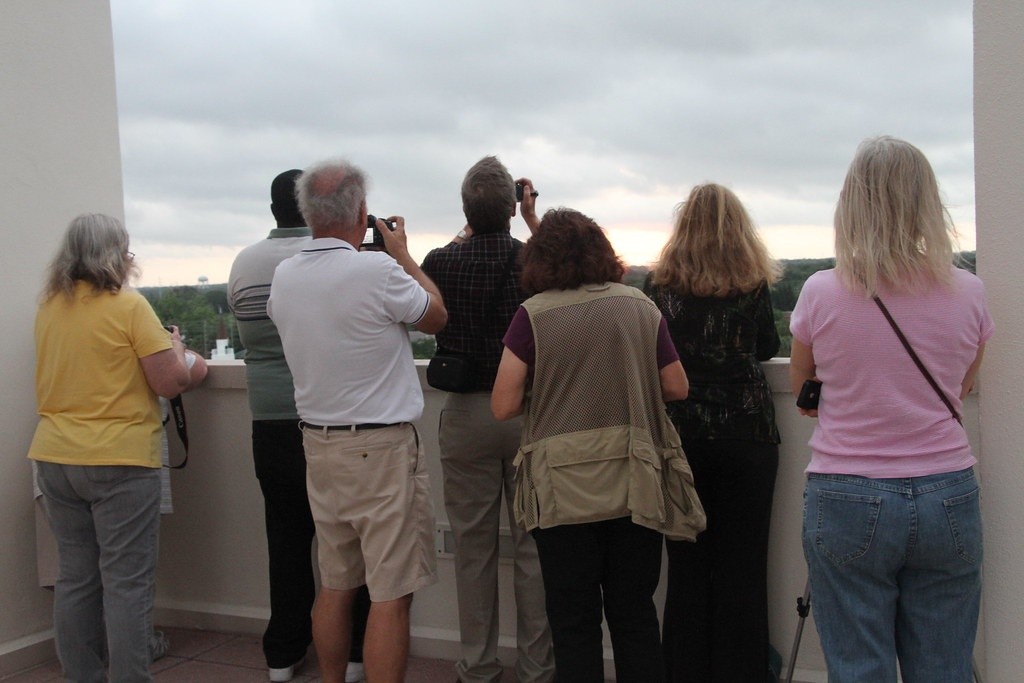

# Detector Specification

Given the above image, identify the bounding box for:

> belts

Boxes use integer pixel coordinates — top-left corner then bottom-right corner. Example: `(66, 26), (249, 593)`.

(301, 422), (407, 430)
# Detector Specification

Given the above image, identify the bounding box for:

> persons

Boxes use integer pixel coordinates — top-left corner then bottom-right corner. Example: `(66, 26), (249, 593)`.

(790, 135), (995, 683)
(644, 183), (781, 683)
(228, 168), (370, 683)
(492, 209), (706, 683)
(266, 159), (448, 683)
(28, 212), (207, 683)
(421, 156), (554, 683)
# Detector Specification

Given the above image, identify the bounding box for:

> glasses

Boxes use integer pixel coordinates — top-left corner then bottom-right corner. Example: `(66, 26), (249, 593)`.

(125, 252), (135, 262)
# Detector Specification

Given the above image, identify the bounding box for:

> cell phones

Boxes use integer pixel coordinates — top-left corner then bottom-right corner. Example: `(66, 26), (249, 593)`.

(796, 380), (822, 409)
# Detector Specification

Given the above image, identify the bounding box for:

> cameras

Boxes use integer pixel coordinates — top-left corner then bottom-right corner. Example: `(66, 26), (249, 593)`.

(361, 214), (394, 247)
(516, 183), (524, 202)
(163, 326), (174, 333)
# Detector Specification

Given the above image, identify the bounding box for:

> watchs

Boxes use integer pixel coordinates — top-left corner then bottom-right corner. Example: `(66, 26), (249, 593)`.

(457, 230), (469, 241)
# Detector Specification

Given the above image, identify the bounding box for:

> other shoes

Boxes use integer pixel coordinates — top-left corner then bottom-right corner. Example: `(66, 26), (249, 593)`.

(345, 662), (364, 682)
(268, 657), (305, 682)
(153, 630), (170, 661)
